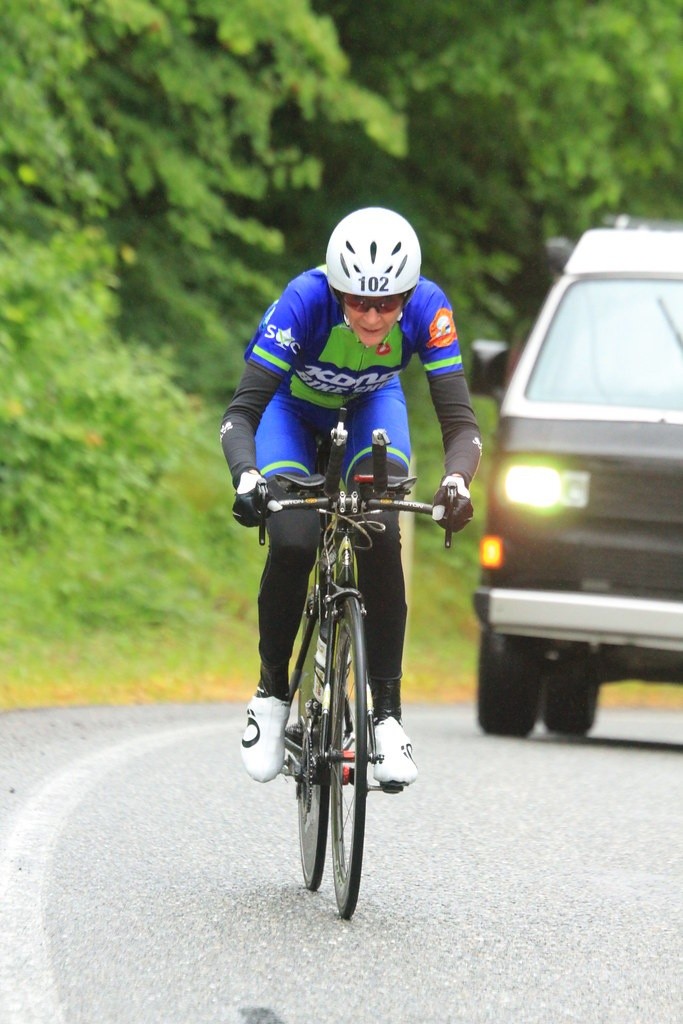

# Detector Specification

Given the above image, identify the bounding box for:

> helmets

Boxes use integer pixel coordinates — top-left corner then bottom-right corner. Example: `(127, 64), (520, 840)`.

(325, 207), (422, 296)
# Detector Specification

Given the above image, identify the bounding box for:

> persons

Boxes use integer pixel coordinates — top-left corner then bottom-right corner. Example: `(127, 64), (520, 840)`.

(220, 206), (483, 784)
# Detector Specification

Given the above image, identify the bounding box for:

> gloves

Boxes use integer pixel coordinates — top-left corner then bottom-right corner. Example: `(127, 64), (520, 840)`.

(230, 469), (272, 528)
(432, 477), (473, 533)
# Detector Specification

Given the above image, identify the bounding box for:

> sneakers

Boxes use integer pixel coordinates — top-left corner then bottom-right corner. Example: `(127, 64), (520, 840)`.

(371, 717), (418, 785)
(240, 696), (292, 784)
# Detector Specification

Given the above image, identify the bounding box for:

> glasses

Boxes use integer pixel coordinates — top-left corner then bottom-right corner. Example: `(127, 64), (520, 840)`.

(338, 291), (410, 313)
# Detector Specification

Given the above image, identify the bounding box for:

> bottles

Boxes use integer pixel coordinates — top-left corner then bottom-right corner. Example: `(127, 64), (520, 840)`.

(312, 620), (351, 705)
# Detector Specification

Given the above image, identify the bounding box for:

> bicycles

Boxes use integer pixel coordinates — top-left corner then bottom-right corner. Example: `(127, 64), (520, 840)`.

(256, 405), (459, 917)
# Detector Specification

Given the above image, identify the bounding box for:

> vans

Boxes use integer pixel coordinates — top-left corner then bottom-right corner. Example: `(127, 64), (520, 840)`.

(470, 227), (683, 736)
